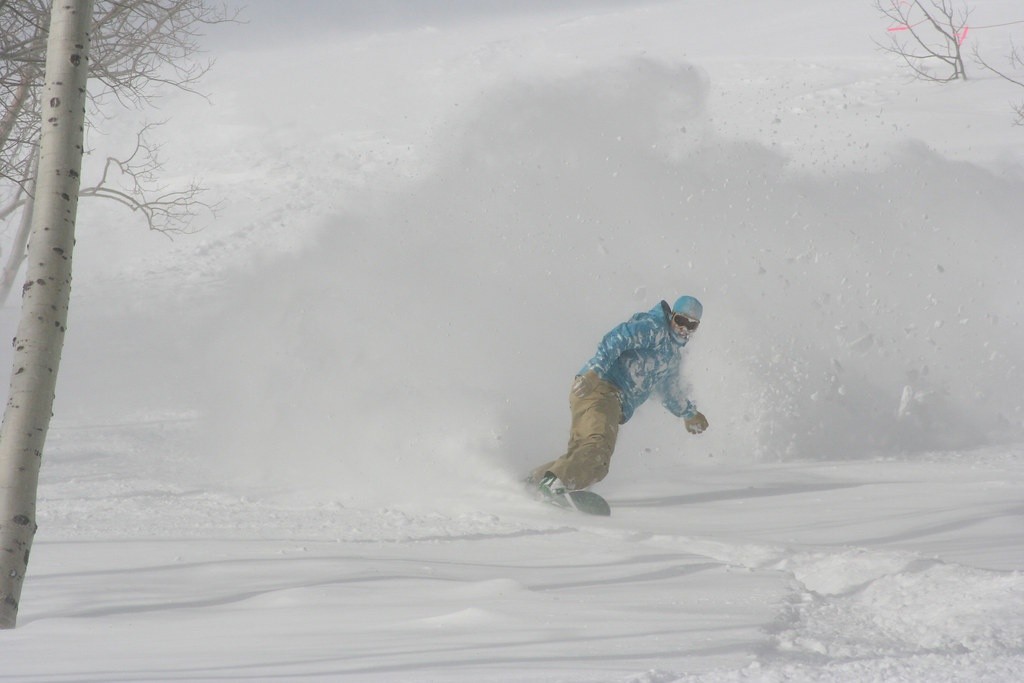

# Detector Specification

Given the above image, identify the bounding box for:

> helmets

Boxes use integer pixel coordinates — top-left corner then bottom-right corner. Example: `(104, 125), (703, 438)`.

(672, 295), (703, 322)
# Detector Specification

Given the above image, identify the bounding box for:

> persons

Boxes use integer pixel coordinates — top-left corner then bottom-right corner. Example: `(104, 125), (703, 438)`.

(522, 294), (709, 516)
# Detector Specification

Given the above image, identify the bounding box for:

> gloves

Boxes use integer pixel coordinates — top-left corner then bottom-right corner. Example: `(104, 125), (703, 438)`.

(684, 412), (709, 435)
(572, 369), (602, 399)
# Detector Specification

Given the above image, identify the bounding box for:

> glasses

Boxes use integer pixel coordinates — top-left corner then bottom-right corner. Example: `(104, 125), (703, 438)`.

(673, 315), (698, 331)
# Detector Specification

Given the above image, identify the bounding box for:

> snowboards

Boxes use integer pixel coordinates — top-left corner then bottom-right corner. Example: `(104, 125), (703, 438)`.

(525, 486), (611, 515)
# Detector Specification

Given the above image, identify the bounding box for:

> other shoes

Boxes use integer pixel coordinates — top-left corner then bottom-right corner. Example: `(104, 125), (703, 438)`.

(539, 475), (565, 501)
